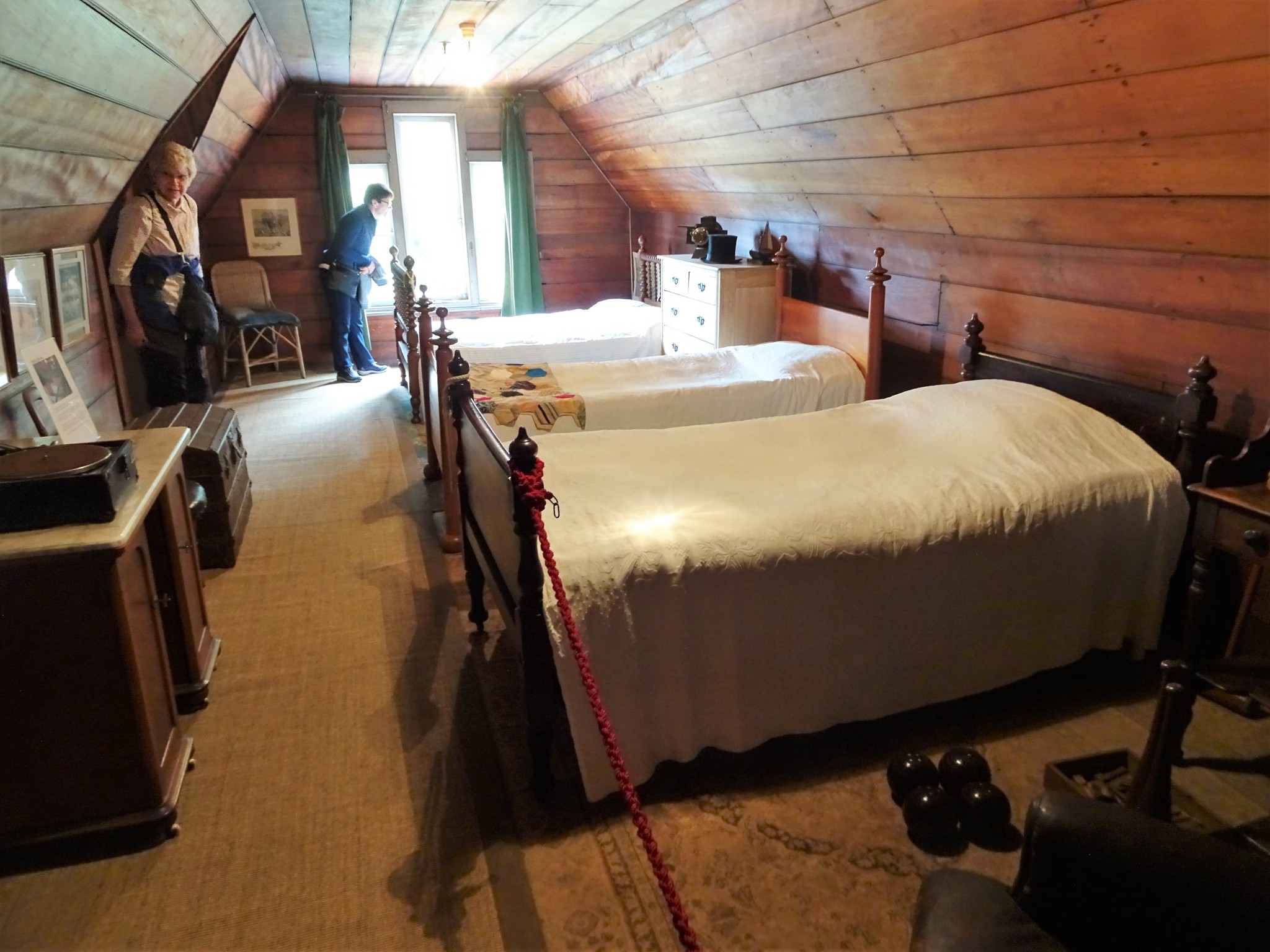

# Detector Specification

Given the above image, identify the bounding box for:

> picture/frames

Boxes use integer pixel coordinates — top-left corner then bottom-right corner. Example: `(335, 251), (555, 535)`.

(0, 246), (92, 393)
(239, 197), (303, 257)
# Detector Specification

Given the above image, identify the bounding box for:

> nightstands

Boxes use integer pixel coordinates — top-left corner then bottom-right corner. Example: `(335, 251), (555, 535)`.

(657, 253), (795, 356)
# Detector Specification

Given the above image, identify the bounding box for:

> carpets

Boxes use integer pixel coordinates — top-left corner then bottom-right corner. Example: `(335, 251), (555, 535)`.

(435, 512), (1270, 952)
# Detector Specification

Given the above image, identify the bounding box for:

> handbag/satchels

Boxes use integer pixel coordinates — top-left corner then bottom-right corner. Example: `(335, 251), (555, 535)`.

(177, 272), (220, 345)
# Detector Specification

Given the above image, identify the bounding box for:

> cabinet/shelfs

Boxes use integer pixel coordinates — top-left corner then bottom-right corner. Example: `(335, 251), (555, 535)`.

(0, 427), (222, 876)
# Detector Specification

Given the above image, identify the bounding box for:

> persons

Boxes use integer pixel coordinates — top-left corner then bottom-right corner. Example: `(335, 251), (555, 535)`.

(319, 184), (395, 383)
(109, 140), (211, 407)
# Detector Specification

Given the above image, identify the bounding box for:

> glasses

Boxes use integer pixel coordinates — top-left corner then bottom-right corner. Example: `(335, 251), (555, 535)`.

(378, 197), (393, 208)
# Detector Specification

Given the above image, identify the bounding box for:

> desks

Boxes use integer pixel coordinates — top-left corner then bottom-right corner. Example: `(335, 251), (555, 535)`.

(1128, 428), (1270, 822)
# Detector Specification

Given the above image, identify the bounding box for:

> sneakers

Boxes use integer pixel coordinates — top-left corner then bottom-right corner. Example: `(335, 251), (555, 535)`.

(357, 361), (387, 376)
(336, 366), (362, 383)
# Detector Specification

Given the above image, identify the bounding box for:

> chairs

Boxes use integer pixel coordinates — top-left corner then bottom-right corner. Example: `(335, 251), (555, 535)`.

(909, 791), (1270, 952)
(212, 260), (306, 387)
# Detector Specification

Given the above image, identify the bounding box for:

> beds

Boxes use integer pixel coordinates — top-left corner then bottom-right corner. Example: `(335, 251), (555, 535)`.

(389, 237), (1219, 840)
